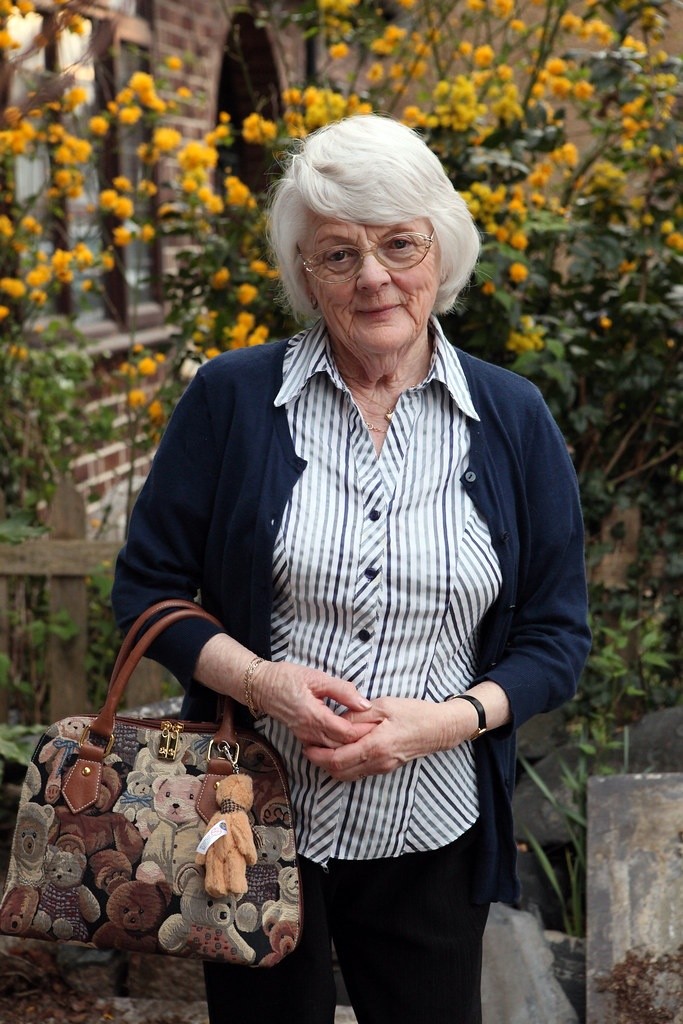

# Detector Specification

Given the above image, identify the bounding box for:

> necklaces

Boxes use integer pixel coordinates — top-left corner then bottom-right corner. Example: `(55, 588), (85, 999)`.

(349, 384), (393, 422)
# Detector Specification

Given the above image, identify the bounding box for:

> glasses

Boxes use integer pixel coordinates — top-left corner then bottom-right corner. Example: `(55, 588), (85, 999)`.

(296, 228), (435, 283)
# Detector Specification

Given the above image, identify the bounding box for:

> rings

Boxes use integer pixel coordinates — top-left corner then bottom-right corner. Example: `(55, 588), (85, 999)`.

(359, 774), (365, 778)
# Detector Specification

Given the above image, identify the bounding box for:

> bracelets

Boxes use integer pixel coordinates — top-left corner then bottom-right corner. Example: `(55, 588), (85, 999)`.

(244, 657), (263, 721)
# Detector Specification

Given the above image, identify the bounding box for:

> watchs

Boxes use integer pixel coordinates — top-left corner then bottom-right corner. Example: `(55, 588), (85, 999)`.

(449, 694), (486, 742)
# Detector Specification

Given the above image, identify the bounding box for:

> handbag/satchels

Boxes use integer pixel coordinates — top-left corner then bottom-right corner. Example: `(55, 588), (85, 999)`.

(0, 598), (304, 969)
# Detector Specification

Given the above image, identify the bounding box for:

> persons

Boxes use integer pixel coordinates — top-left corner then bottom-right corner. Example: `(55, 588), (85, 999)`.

(113, 115), (593, 1024)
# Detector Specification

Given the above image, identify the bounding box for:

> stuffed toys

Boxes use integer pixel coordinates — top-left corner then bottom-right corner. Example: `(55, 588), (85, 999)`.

(195, 773), (257, 897)
